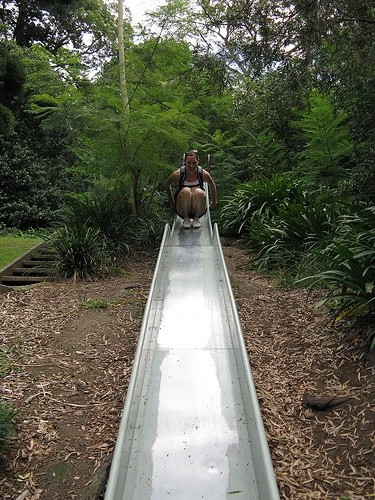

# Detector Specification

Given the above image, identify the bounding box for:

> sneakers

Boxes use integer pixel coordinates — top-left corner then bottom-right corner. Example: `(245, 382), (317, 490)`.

(182, 217), (192, 228)
(192, 216), (201, 227)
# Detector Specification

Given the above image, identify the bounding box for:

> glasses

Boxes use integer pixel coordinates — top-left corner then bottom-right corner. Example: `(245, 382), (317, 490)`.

(186, 149), (197, 153)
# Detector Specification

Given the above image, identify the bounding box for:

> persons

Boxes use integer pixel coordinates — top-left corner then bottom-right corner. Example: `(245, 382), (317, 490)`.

(166, 150), (218, 229)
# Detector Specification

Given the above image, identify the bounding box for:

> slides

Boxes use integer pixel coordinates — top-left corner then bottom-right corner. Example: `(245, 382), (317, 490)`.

(101, 150), (283, 500)
